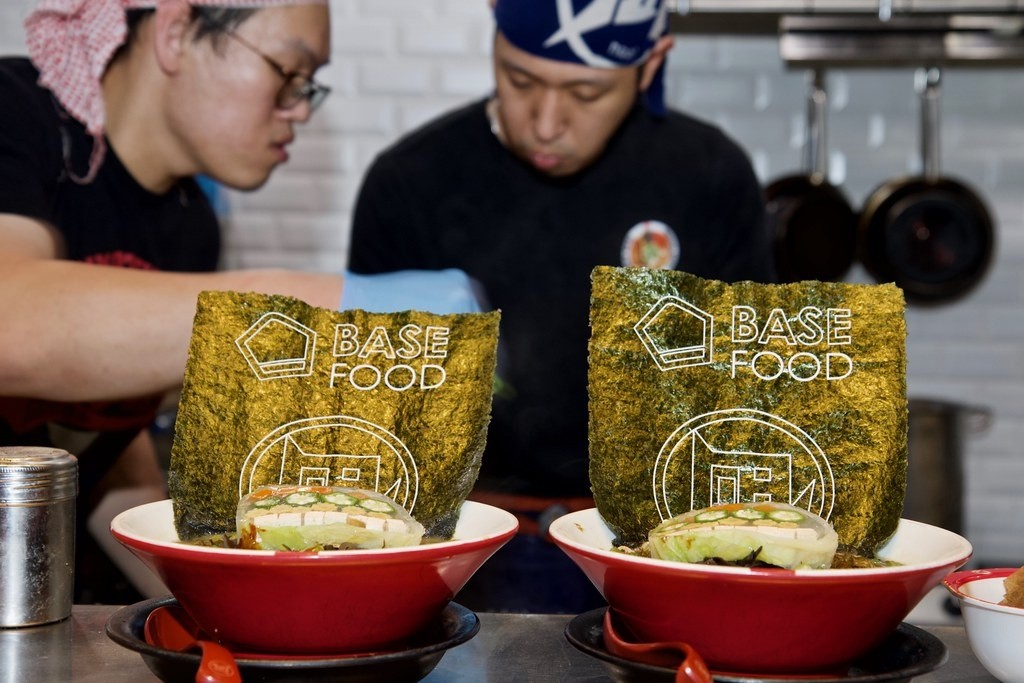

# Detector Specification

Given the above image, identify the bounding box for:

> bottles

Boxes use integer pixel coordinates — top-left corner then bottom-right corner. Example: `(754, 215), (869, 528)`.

(0, 445), (78, 628)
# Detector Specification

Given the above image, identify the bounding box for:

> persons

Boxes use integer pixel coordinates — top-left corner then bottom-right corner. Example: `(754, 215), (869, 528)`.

(0, 0), (484, 606)
(348, 0), (780, 615)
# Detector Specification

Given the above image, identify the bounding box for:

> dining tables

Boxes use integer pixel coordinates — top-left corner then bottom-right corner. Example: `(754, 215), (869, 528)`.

(0, 604), (1003, 683)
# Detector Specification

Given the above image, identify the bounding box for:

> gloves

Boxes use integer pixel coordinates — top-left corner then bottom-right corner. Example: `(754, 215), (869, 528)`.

(335, 267), (485, 314)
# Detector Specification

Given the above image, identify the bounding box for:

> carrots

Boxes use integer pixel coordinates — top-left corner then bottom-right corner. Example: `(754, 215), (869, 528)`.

(755, 505), (776, 512)
(249, 487), (368, 502)
(706, 504), (744, 511)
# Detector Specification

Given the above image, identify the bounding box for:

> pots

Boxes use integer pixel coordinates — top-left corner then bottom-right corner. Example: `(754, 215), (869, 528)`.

(751, 65), (856, 289)
(858, 69), (994, 307)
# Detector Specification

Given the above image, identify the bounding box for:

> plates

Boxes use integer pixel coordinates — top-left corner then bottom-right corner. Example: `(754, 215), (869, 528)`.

(563, 603), (951, 683)
(104, 593), (480, 683)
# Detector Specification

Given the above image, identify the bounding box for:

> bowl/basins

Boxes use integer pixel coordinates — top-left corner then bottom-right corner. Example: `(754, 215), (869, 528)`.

(109, 495), (520, 656)
(549, 505), (973, 675)
(941, 560), (1024, 683)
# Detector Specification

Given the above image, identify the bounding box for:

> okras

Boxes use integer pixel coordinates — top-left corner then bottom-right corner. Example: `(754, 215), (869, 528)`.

(661, 508), (804, 529)
(255, 491), (396, 514)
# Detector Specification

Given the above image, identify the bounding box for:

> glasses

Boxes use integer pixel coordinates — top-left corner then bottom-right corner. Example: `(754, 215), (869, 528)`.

(198, 8), (330, 112)
